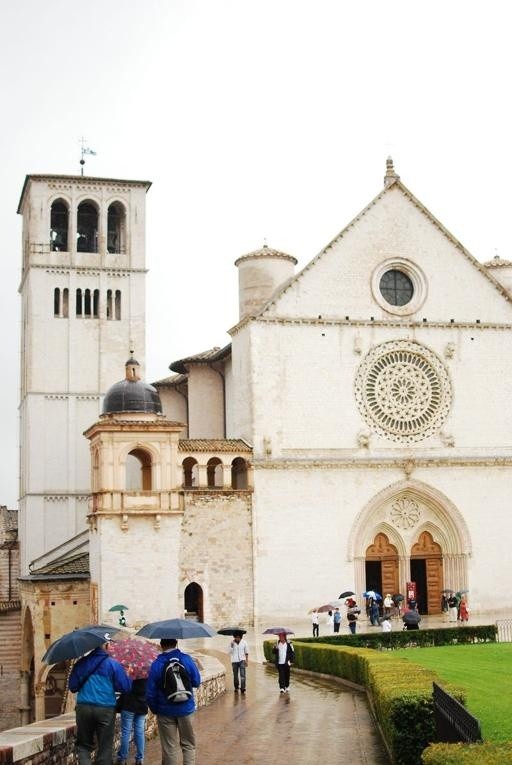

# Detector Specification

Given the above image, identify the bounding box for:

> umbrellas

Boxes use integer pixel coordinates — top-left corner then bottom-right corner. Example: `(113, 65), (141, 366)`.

(105, 638), (162, 681)
(42, 624), (122, 666)
(134, 619), (217, 651)
(109, 605), (129, 612)
(262, 626), (295, 636)
(217, 626), (247, 636)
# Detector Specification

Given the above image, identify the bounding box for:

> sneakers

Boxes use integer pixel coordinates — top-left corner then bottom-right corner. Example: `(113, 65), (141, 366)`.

(135, 761), (144, 765)
(280, 688), (289, 694)
(117, 759), (128, 765)
(234, 688), (246, 693)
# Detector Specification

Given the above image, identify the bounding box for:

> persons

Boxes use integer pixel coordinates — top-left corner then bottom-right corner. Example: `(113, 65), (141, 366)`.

(230, 632), (249, 694)
(272, 633), (296, 694)
(119, 611), (127, 627)
(145, 639), (202, 765)
(68, 641), (134, 765)
(112, 678), (150, 765)
(308, 585), (471, 637)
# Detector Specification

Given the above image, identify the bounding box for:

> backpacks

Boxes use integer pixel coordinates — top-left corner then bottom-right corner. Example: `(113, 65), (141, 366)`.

(162, 653), (193, 704)
